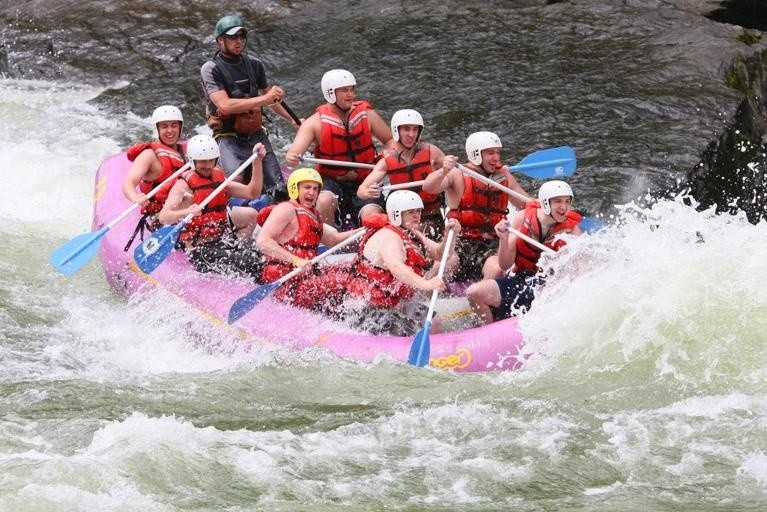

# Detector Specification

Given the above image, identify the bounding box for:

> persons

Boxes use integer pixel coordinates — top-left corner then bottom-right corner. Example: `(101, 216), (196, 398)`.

(358, 109), (450, 244)
(467, 180), (584, 324)
(339, 189), (462, 338)
(200, 15), (309, 199)
(284, 70), (393, 234)
(257, 167), (359, 324)
(423, 132), (533, 283)
(160, 135), (269, 251)
(124, 105), (194, 255)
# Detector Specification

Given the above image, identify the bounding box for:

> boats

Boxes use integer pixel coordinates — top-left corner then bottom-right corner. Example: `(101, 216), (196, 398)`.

(92, 137), (596, 375)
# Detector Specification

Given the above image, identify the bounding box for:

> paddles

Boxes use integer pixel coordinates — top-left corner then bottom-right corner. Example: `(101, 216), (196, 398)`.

(227, 230), (370, 326)
(134, 152), (260, 274)
(49, 162), (193, 277)
(454, 162), (604, 236)
(407, 227), (454, 367)
(371, 146), (578, 191)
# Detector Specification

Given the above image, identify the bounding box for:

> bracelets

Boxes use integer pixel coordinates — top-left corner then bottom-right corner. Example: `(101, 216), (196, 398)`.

(293, 256), (299, 268)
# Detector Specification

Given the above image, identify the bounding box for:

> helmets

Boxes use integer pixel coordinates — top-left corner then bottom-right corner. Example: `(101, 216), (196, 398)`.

(391, 108), (425, 143)
(287, 167), (323, 200)
(215, 14), (249, 43)
(187, 134), (220, 171)
(465, 131), (503, 166)
(320, 69), (358, 104)
(151, 104), (185, 139)
(538, 179), (574, 215)
(386, 189), (425, 226)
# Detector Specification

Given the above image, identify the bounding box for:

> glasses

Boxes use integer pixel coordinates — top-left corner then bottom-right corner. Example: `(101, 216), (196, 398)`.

(223, 33), (243, 39)
